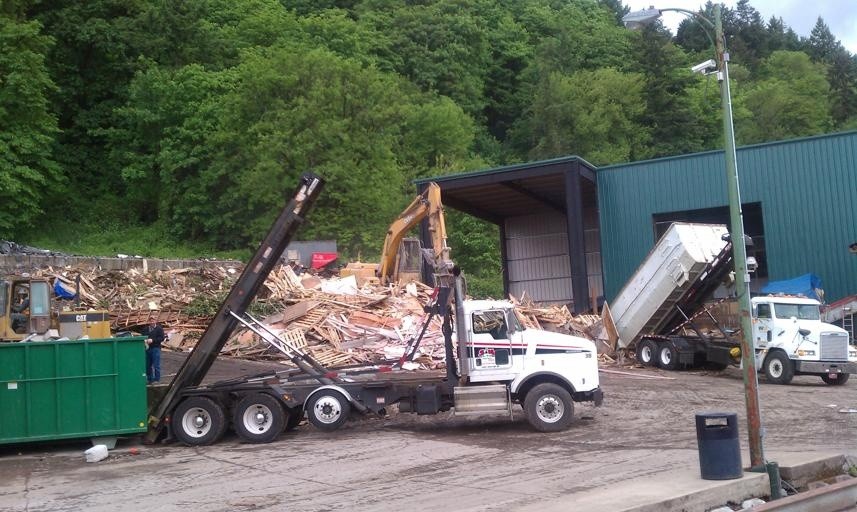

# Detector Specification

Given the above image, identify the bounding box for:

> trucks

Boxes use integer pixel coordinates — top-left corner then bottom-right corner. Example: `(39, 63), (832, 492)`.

(602, 219), (857, 387)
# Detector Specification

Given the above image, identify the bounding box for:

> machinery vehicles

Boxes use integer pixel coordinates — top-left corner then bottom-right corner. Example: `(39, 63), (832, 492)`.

(336, 181), (469, 304)
(0, 274), (117, 345)
(135, 169), (605, 446)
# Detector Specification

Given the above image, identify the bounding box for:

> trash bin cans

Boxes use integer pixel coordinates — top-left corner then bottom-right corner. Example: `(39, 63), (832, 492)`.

(696, 413), (742, 479)
(0, 336), (148, 449)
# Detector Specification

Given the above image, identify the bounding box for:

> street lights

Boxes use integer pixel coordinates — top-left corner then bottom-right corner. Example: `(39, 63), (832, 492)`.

(620, 7), (771, 474)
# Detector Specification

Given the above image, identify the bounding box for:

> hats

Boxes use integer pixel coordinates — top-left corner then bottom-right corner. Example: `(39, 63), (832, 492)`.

(150, 315), (156, 320)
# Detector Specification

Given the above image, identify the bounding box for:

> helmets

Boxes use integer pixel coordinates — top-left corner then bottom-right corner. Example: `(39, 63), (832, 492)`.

(18, 288), (28, 294)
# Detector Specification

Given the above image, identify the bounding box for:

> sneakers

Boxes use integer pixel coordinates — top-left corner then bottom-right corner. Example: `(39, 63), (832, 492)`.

(146, 381), (159, 385)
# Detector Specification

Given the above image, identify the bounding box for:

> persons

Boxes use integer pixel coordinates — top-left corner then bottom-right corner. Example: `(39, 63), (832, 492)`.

(9, 287), (30, 333)
(141, 313), (166, 385)
